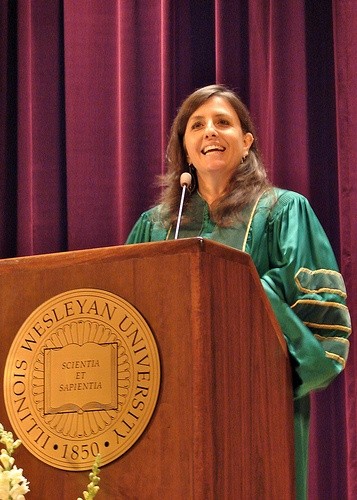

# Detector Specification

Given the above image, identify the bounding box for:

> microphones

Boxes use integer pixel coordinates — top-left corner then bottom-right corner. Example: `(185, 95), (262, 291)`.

(174, 172), (192, 239)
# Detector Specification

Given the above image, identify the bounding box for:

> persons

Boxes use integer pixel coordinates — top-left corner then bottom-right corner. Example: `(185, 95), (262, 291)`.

(124, 84), (353, 500)
